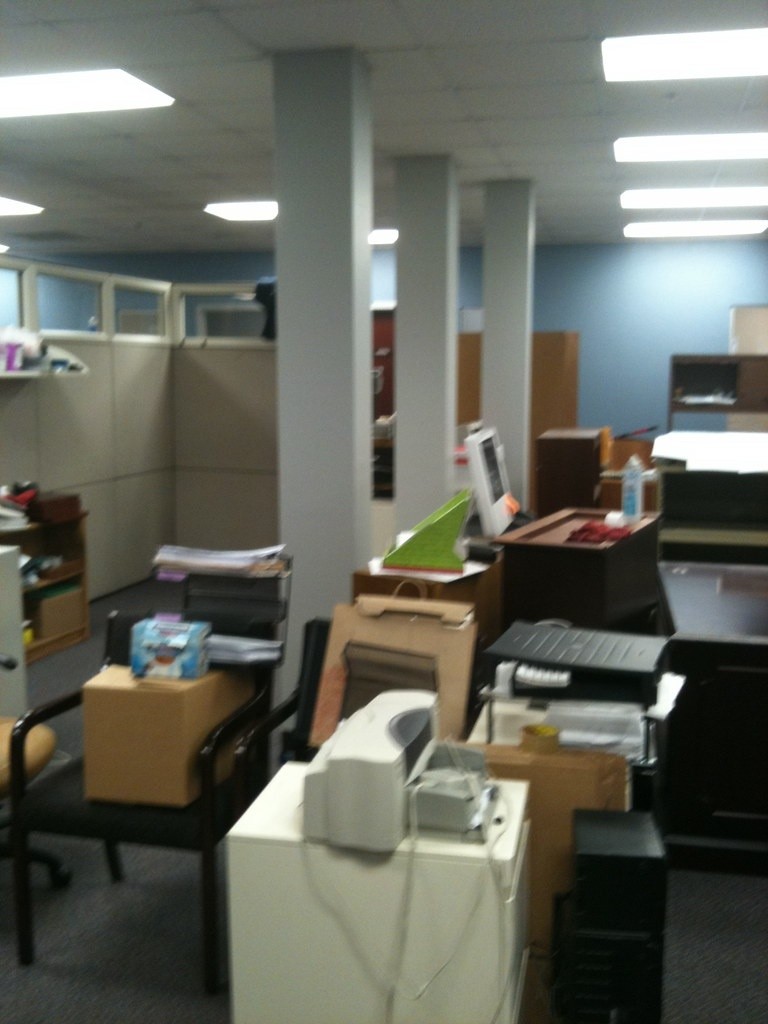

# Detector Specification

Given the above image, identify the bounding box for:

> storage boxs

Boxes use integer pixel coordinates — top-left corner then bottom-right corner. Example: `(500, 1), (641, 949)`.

(459, 701), (657, 810)
(468, 693), (626, 938)
(82, 664), (252, 807)
(35, 588), (83, 638)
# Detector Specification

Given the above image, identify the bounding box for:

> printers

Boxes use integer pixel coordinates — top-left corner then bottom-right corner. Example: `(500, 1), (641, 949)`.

(300, 685), (500, 852)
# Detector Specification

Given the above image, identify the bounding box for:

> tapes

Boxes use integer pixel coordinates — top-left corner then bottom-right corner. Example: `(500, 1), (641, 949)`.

(519, 723), (560, 754)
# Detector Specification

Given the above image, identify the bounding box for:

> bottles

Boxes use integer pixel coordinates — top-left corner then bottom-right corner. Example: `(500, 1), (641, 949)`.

(621, 454), (644, 524)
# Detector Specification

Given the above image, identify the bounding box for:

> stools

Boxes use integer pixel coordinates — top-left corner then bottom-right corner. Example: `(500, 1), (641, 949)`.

(0, 715), (73, 892)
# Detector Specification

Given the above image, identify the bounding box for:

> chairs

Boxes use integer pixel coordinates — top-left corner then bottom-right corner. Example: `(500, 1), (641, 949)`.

(9, 611), (275, 992)
(234, 619), (329, 911)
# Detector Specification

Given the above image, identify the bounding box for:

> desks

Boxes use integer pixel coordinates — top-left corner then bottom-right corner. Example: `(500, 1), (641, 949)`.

(658, 558), (768, 876)
(353, 557), (501, 650)
(496, 508), (662, 631)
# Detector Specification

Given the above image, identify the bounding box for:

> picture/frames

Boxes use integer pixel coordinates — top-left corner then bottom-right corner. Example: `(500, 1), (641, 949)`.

(465, 426), (513, 535)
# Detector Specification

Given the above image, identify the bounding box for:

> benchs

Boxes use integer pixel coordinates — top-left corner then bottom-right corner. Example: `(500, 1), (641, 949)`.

(657, 470), (768, 561)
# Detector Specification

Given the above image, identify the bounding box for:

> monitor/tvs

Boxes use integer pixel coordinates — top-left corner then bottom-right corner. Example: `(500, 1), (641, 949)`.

(465, 428), (513, 539)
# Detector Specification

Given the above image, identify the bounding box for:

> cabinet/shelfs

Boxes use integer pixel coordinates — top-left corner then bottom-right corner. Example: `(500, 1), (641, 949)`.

(0, 511), (90, 661)
(226, 761), (531, 1024)
(536, 428), (603, 519)
